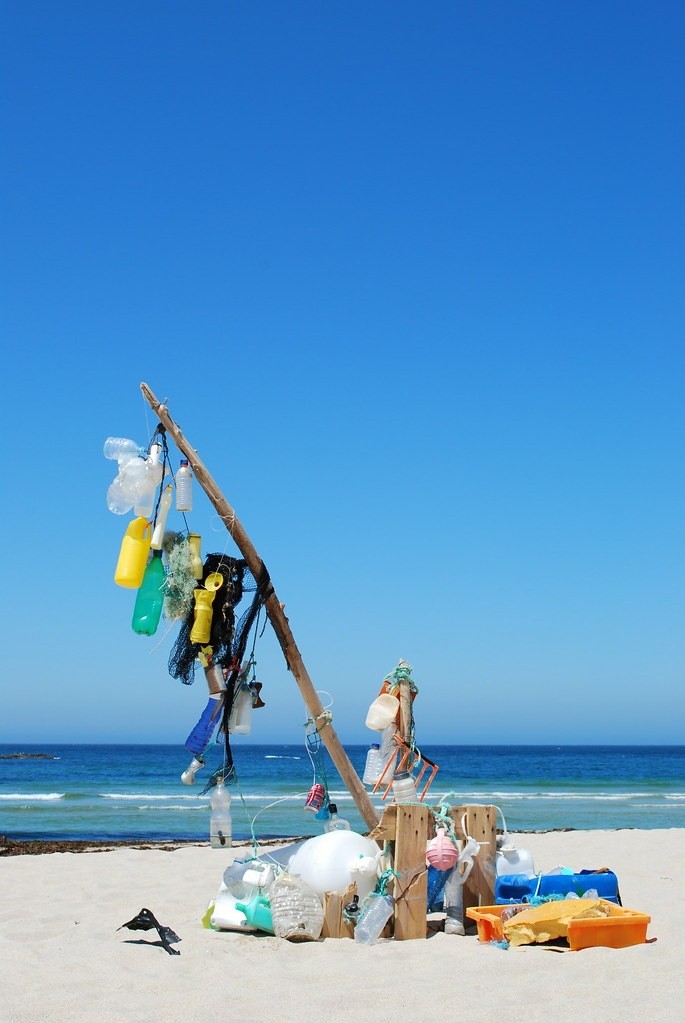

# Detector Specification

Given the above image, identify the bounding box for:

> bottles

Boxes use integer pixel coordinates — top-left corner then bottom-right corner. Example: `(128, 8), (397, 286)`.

(104, 437), (217, 644)
(363, 726), (399, 786)
(426, 864), (454, 913)
(305, 784), (325, 813)
(365, 685), (400, 730)
(235, 667), (259, 734)
(210, 856), (274, 932)
(185, 699), (224, 754)
(392, 770), (418, 803)
(180, 755), (206, 785)
(445, 872), (465, 936)
(324, 804), (350, 833)
(210, 776), (232, 849)
(496, 843), (535, 877)
(315, 794), (330, 819)
(289, 830), (380, 902)
(234, 897), (274, 934)
(269, 878), (325, 942)
(343, 895), (395, 946)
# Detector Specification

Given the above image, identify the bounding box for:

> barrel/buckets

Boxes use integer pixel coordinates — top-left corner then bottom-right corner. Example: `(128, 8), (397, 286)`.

(496, 869), (618, 904)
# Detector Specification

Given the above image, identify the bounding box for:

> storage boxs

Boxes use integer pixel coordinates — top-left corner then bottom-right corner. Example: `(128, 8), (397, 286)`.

(466, 899), (650, 950)
